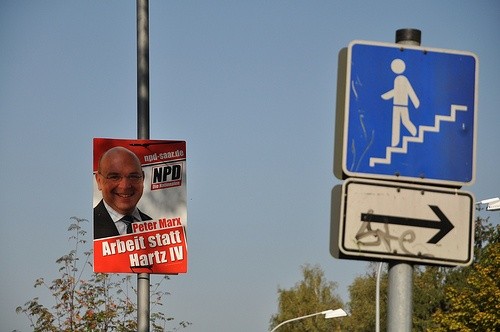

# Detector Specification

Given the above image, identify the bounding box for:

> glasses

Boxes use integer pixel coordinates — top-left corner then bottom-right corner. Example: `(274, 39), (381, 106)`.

(100, 172), (145, 183)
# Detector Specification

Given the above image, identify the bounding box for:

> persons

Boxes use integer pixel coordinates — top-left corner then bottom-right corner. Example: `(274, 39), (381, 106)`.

(94, 147), (154, 240)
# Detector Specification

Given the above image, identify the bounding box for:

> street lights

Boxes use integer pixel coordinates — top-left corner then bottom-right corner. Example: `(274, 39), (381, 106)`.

(269, 307), (348, 332)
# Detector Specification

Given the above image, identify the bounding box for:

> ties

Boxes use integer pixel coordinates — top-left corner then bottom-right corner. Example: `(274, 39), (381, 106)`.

(120, 215), (135, 234)
